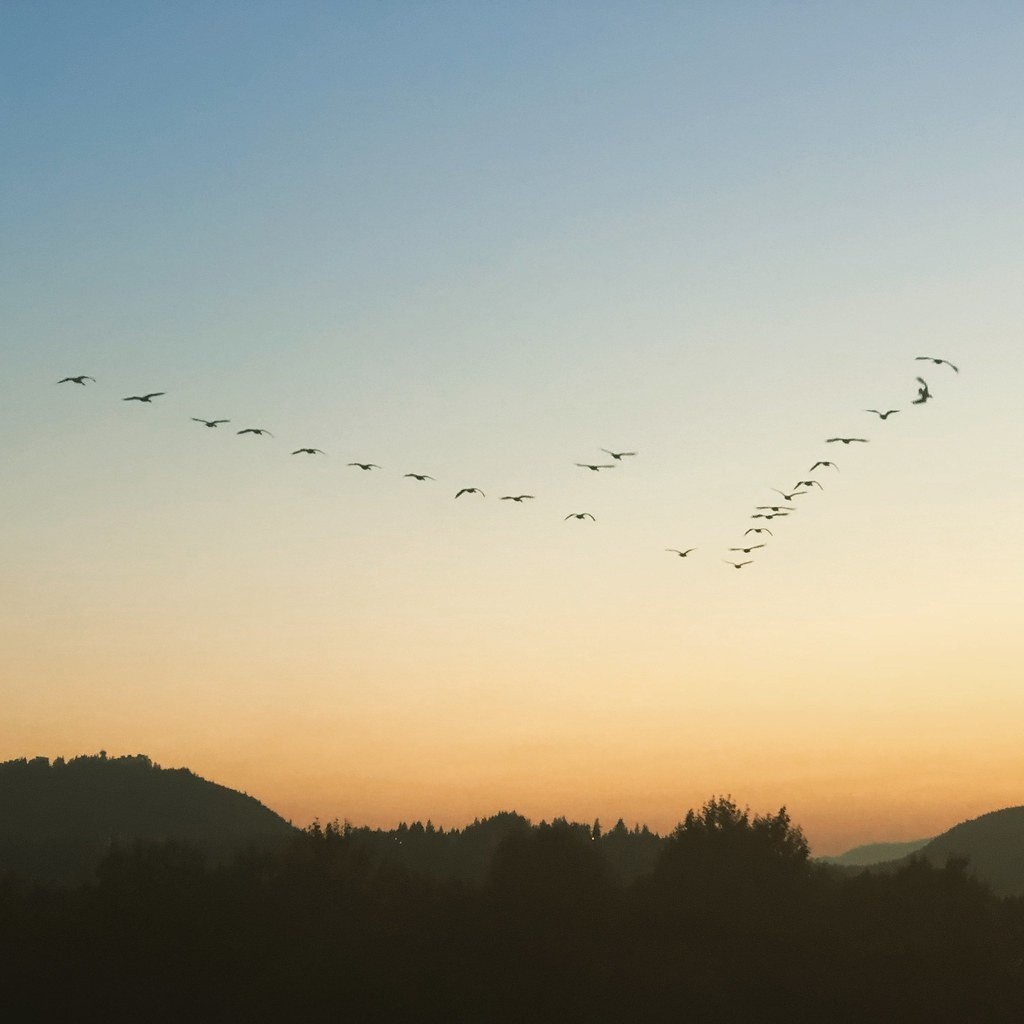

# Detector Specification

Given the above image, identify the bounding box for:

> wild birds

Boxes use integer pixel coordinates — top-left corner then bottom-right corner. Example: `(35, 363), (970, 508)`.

(56, 376), (693, 558)
(723, 357), (958, 567)
